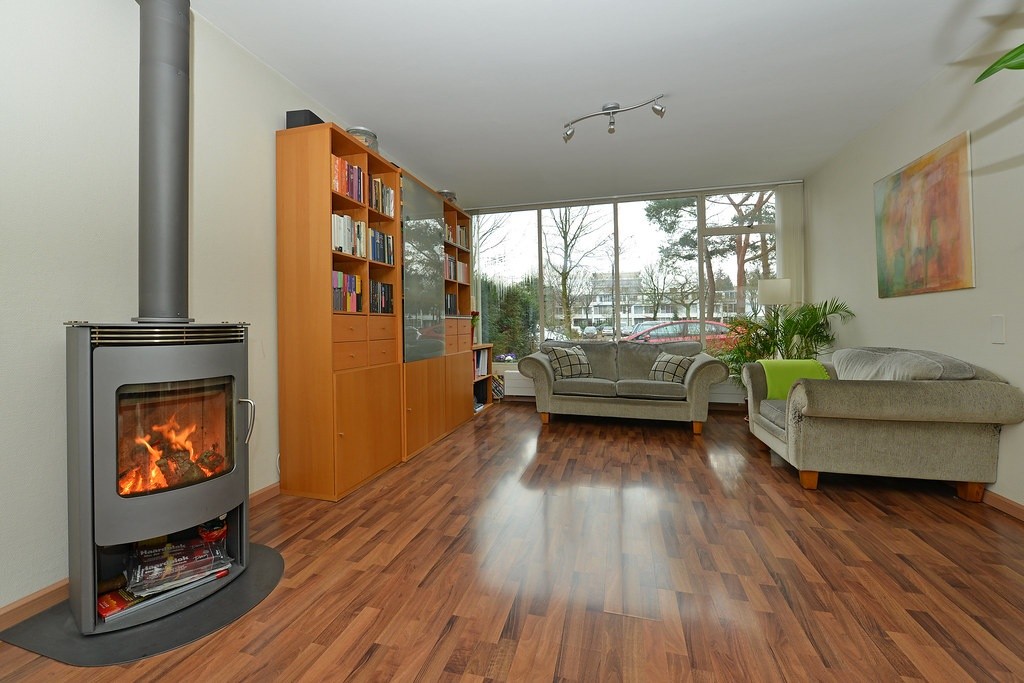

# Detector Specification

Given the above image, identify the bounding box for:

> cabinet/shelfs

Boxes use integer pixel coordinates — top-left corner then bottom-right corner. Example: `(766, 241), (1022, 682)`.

(275, 122), (472, 503)
(472, 344), (493, 415)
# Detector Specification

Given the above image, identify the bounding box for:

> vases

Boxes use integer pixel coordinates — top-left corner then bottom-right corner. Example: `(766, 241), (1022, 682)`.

(471, 327), (474, 346)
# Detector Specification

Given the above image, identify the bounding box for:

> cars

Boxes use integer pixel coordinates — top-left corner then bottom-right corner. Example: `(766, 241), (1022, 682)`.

(571, 326), (583, 337)
(621, 319), (756, 355)
(583, 326), (598, 338)
(533, 324), (570, 350)
(602, 326), (613, 337)
(621, 322), (666, 336)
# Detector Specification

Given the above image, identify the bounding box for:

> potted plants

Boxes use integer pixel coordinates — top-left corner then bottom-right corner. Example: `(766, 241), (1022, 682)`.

(703, 296), (856, 418)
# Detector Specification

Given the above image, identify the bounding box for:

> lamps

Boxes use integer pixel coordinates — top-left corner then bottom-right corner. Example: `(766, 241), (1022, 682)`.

(563, 93), (666, 144)
(758, 279), (792, 359)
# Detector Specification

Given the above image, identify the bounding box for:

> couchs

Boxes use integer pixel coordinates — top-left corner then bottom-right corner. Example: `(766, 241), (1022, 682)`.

(518, 338), (730, 434)
(741, 346), (1024, 503)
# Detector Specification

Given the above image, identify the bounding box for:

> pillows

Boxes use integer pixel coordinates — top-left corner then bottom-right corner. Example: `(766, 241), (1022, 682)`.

(648, 351), (695, 384)
(548, 345), (592, 380)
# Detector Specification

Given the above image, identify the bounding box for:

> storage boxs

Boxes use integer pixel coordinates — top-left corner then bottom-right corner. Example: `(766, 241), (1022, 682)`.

(286, 109), (325, 129)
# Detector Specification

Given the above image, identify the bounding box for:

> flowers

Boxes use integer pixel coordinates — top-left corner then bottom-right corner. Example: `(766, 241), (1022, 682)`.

(471, 311), (480, 328)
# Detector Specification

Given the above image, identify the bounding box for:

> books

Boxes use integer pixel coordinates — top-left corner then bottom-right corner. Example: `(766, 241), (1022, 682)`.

(445, 253), (456, 280)
(369, 280), (394, 314)
(331, 154), (363, 203)
(368, 174), (395, 218)
(332, 271), (362, 312)
(457, 261), (469, 283)
(445, 223), (454, 242)
(473, 349), (488, 380)
(332, 214), (366, 258)
(368, 228), (394, 265)
(445, 293), (457, 316)
(457, 225), (468, 248)
